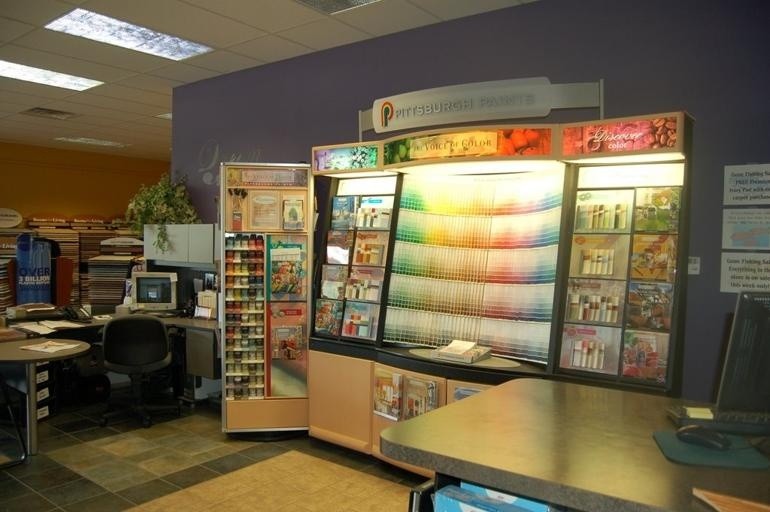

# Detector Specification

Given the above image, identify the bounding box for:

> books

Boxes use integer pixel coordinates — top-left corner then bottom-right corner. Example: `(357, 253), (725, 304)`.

(432, 340), (492, 364)
(560, 203), (627, 370)
(373, 367), (439, 422)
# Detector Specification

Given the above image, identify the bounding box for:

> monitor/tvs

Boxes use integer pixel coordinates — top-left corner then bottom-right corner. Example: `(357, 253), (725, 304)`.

(131, 272), (177, 315)
(716, 289), (770, 413)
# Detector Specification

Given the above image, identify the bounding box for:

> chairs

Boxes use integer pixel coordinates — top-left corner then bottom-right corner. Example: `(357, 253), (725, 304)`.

(98, 316), (181, 429)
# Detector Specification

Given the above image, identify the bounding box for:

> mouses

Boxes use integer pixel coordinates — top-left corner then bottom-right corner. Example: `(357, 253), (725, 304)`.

(675, 424), (731, 450)
(168, 328), (178, 335)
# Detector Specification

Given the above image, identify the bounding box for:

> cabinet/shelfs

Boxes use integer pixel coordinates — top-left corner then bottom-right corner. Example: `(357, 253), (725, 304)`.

(307, 163), (685, 496)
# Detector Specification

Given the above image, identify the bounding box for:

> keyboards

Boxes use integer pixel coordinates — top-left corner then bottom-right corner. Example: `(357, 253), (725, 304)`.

(663, 404), (770, 435)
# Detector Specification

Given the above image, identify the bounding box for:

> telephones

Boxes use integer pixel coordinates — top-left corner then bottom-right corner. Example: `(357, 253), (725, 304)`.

(65, 306), (92, 321)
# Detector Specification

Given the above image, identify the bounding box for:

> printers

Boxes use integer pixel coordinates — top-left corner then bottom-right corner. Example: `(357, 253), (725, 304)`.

(6, 303), (65, 320)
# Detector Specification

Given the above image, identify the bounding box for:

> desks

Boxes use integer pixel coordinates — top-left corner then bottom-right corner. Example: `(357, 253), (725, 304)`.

(11, 306), (221, 422)
(377, 376), (767, 511)
(1, 336), (89, 458)
